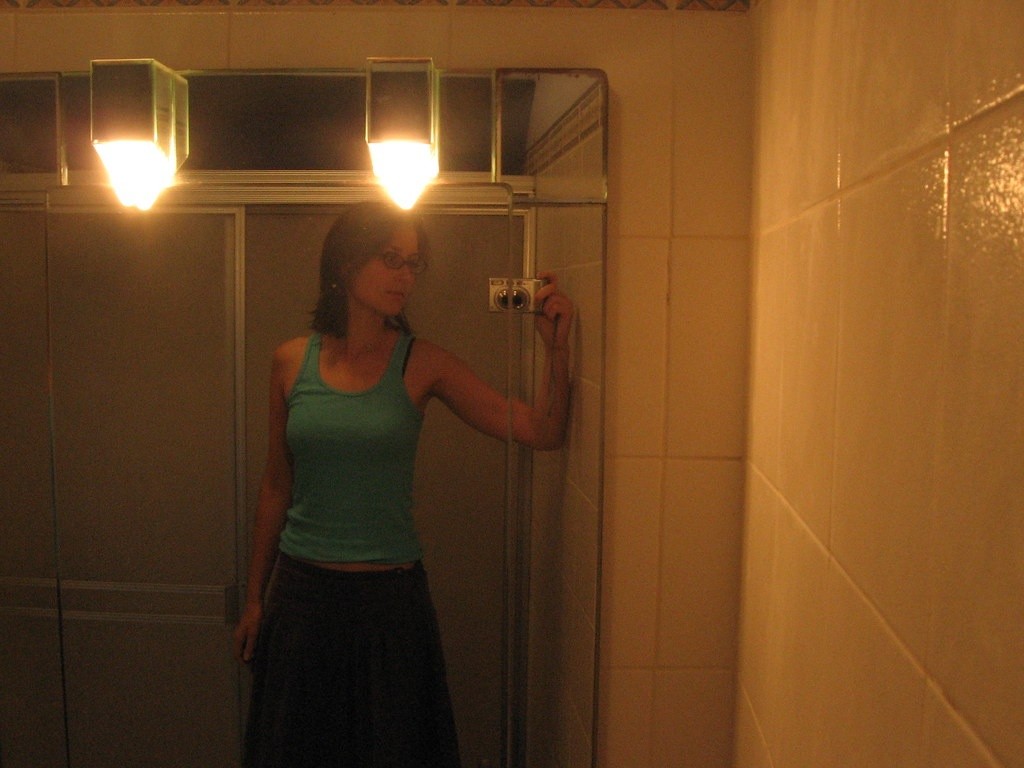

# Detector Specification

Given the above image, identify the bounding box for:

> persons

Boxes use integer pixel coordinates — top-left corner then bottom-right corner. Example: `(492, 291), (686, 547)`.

(233, 204), (575, 768)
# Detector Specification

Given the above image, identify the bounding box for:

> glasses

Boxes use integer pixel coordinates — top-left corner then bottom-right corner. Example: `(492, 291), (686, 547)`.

(378, 251), (429, 275)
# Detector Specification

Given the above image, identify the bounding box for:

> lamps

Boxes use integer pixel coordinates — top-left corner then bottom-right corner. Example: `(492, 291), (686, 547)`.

(365, 55), (441, 195)
(93, 56), (175, 207)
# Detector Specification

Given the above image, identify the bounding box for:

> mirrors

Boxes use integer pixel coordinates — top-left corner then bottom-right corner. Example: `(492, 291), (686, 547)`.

(0, 68), (608, 768)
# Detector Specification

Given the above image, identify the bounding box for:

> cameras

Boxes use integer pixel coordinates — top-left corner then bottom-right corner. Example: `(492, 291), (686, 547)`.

(489, 277), (549, 314)
